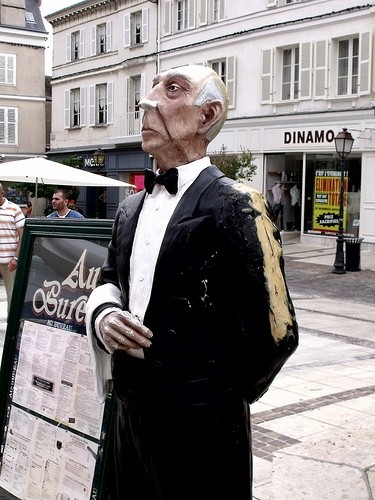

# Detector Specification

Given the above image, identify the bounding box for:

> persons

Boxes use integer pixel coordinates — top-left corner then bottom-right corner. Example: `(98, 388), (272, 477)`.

(272, 184), (285, 232)
(91, 191), (107, 219)
(0, 183), (25, 314)
(68, 194), (76, 210)
(87, 66), (299, 500)
(290, 185), (301, 231)
(25, 202), (32, 218)
(46, 189), (86, 219)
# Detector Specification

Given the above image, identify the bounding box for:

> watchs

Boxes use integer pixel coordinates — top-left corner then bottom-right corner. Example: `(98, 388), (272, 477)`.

(14, 257), (18, 261)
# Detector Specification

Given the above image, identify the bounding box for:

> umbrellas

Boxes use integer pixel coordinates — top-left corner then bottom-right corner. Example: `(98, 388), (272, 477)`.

(0, 157), (138, 187)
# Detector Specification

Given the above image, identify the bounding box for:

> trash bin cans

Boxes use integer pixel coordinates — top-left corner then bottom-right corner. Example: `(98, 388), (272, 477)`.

(344, 237), (365, 274)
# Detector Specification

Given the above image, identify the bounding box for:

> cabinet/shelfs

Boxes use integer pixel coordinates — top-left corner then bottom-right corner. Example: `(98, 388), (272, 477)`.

(267, 181), (300, 191)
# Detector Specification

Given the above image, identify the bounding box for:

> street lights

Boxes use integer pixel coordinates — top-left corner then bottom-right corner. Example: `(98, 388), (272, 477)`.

(90, 146), (105, 219)
(333, 127), (355, 274)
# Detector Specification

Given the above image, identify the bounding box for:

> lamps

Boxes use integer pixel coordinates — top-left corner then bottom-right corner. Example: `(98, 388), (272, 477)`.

(93, 148), (105, 164)
